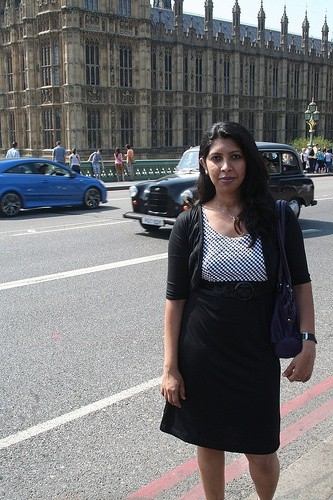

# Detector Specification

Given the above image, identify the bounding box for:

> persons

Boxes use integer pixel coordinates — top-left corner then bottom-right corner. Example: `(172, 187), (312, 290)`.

(114, 148), (124, 181)
(69, 148), (81, 174)
(159, 120), (316, 500)
(125, 144), (135, 180)
(283, 143), (333, 174)
(6, 142), (20, 158)
(88, 149), (104, 180)
(52, 141), (66, 172)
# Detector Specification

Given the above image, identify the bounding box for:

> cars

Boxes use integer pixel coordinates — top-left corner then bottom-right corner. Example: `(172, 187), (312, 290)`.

(0, 157), (108, 218)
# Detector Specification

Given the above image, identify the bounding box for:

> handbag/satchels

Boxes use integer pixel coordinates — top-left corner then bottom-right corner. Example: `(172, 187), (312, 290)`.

(268, 199), (304, 357)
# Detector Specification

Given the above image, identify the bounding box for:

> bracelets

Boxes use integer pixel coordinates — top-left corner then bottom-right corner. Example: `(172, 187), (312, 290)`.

(301, 332), (317, 343)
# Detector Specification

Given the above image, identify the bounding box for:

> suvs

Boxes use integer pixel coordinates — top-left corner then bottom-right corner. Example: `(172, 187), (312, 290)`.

(122, 141), (318, 232)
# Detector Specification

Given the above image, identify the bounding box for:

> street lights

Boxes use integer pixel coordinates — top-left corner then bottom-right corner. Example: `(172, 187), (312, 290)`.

(304, 96), (321, 147)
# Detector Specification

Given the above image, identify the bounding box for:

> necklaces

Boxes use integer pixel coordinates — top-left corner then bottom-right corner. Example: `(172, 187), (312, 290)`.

(214, 202), (235, 221)
